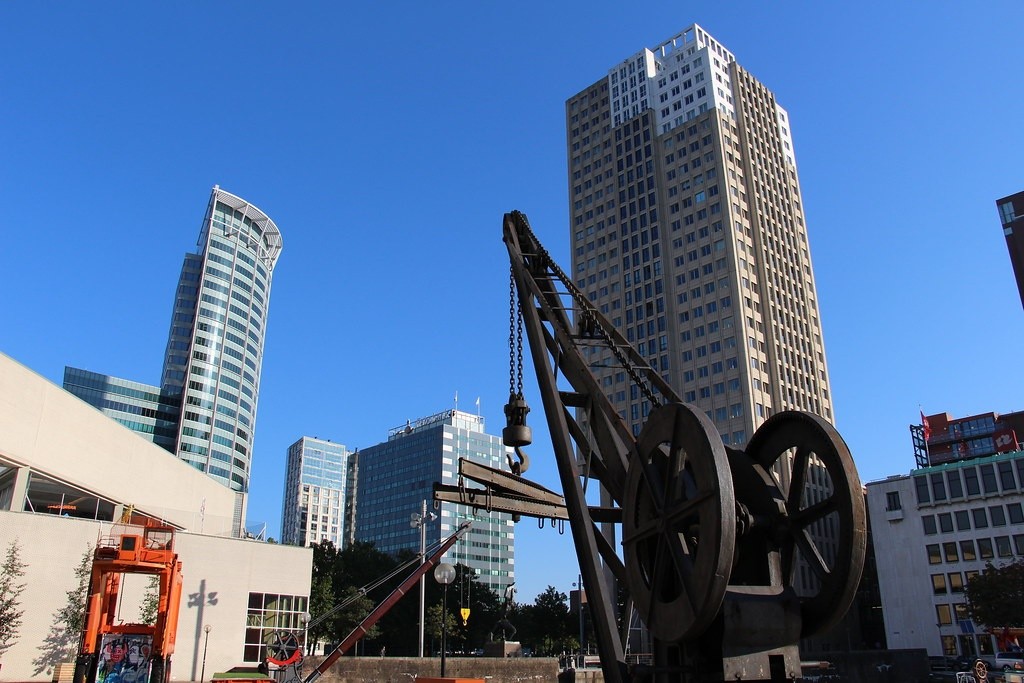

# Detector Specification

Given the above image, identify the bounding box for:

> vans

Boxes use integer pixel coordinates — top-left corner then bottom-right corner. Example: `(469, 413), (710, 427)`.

(994, 651), (1024, 672)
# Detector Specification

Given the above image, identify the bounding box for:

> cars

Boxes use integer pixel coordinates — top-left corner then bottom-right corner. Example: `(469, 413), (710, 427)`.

(927, 654), (993, 672)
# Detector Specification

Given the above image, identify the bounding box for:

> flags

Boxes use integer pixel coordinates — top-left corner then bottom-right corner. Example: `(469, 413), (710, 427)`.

(921, 412), (930, 441)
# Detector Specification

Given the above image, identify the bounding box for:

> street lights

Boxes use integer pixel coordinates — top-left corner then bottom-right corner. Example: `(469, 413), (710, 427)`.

(434, 564), (456, 676)
(410, 499), (438, 657)
